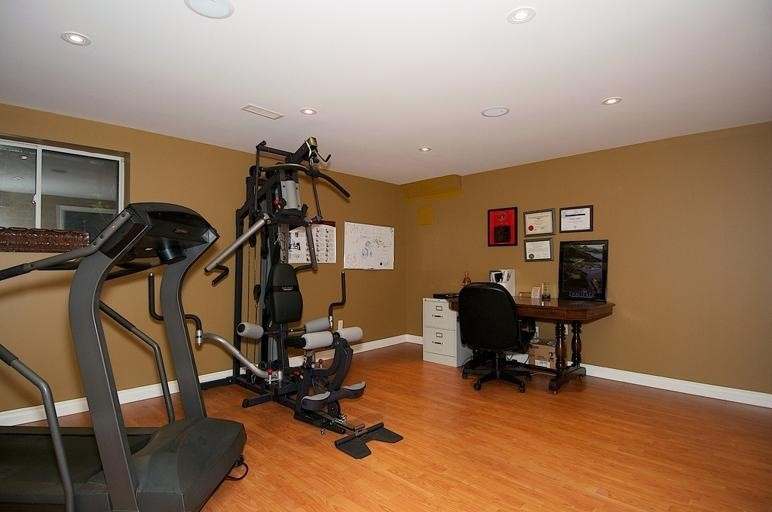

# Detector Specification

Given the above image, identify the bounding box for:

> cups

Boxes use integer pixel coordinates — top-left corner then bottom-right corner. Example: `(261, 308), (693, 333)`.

(541, 281), (552, 301)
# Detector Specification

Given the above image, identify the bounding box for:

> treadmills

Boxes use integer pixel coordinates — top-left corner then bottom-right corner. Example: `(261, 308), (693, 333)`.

(0, 202), (247, 512)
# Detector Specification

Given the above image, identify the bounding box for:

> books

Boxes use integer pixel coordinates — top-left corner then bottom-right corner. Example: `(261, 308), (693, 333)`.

(489, 268), (511, 284)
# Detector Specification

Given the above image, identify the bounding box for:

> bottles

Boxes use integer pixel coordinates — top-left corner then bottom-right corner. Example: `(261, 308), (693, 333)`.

(461, 272), (472, 289)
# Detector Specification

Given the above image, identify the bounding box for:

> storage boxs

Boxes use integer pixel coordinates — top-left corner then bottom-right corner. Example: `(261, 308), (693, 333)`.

(528, 339), (557, 376)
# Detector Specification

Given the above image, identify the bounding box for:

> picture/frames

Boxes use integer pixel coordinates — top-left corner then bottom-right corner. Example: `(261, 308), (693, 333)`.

(559, 240), (609, 302)
(488, 207), (517, 246)
(523, 208), (556, 237)
(524, 237), (554, 262)
(560, 205), (593, 233)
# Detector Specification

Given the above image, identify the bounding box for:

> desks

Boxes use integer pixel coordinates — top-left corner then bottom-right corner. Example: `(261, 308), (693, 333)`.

(449, 297), (616, 395)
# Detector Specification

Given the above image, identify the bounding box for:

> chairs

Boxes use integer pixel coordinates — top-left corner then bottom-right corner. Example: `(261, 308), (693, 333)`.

(457, 282), (535, 393)
